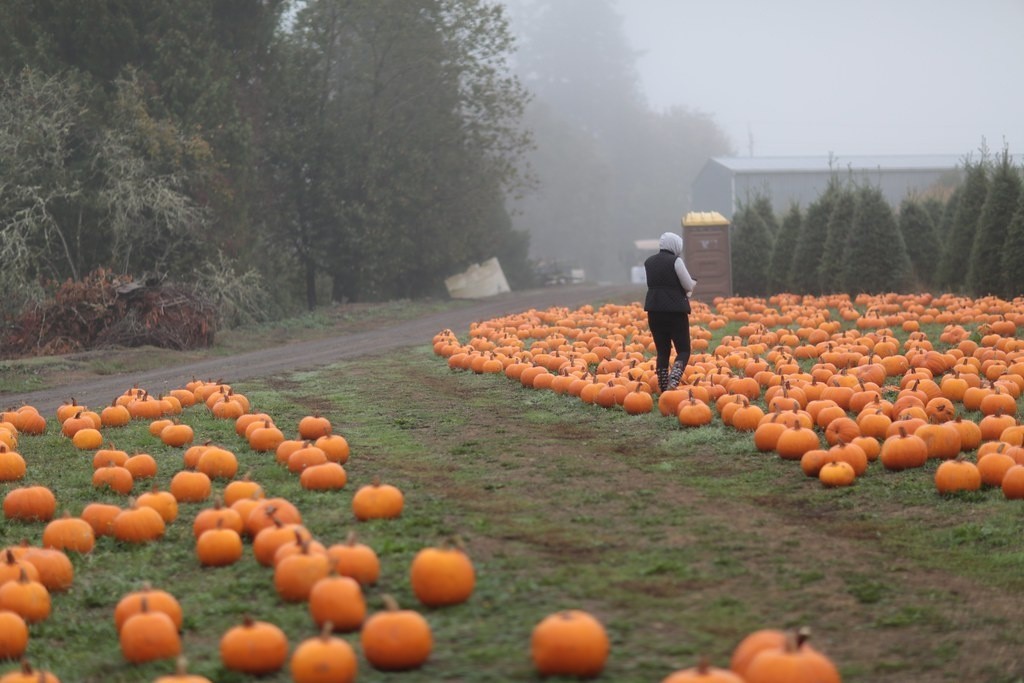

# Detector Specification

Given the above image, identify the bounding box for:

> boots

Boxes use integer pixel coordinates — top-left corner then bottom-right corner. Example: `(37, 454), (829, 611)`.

(670, 360), (685, 392)
(656, 365), (667, 393)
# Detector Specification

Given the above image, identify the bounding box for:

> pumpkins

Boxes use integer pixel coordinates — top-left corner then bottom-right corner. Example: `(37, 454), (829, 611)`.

(0, 377), (842, 683)
(433, 288), (1024, 497)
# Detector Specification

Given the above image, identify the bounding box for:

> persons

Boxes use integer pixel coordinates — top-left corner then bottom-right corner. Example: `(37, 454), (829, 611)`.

(644, 232), (696, 393)
